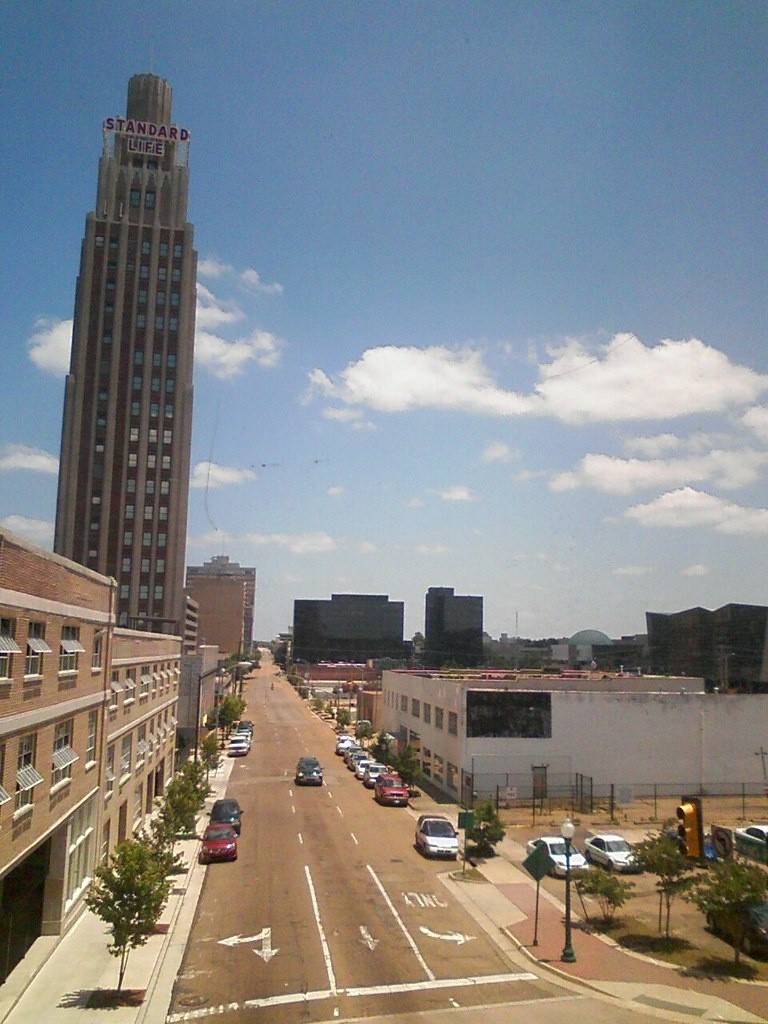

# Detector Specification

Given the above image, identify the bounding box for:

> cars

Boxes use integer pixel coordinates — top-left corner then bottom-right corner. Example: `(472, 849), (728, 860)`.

(336, 735), (368, 770)
(237, 720), (255, 745)
(296, 756), (324, 787)
(364, 762), (389, 788)
(205, 797), (244, 835)
(355, 759), (374, 781)
(198, 822), (239, 863)
(736, 824), (768, 843)
(707, 897), (768, 956)
(527, 835), (590, 880)
(229, 736), (251, 758)
(415, 815), (459, 860)
(584, 833), (646, 872)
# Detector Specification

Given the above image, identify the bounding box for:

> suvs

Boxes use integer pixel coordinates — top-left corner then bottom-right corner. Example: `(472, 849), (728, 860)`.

(374, 773), (409, 808)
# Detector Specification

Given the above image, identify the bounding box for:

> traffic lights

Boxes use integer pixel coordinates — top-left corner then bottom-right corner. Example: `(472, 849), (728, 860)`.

(676, 795), (705, 863)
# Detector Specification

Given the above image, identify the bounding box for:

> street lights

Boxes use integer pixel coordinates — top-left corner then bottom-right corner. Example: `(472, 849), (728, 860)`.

(560, 817), (580, 965)
